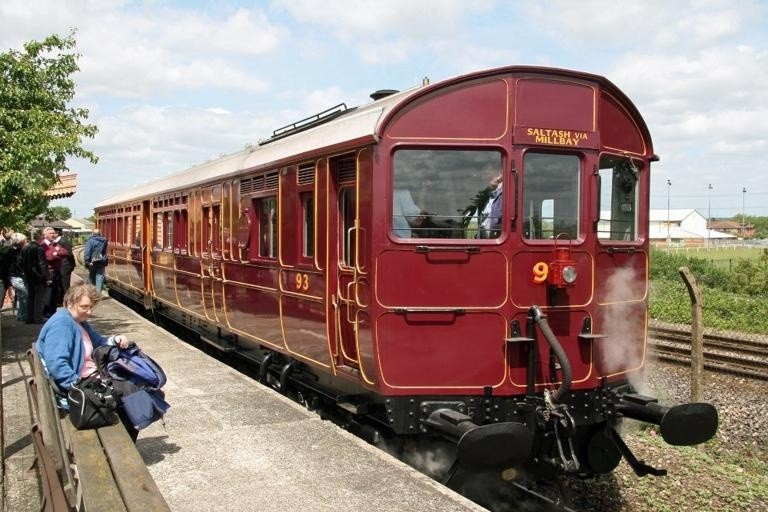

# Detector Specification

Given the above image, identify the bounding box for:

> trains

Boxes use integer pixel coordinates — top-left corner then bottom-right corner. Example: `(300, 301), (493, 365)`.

(83, 60), (719, 497)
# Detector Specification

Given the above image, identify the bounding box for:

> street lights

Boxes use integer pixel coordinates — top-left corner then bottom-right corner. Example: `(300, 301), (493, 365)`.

(741, 187), (747, 229)
(707, 182), (714, 246)
(665, 178), (672, 238)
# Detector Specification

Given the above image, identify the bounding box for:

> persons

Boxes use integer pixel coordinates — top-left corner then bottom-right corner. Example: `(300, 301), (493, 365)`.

(0, 224), (109, 325)
(392, 157), (435, 239)
(34, 280), (169, 445)
(477, 156), (504, 238)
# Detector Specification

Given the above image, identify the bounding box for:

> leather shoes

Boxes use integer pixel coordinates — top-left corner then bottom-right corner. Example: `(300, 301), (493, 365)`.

(26, 318), (46, 324)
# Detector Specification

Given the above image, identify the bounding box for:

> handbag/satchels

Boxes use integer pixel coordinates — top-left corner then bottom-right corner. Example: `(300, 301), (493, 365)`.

(67, 377), (114, 431)
(90, 247), (102, 265)
(92, 341), (171, 430)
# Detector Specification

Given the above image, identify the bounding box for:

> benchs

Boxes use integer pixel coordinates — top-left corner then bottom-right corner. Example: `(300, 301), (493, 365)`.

(26, 340), (173, 512)
